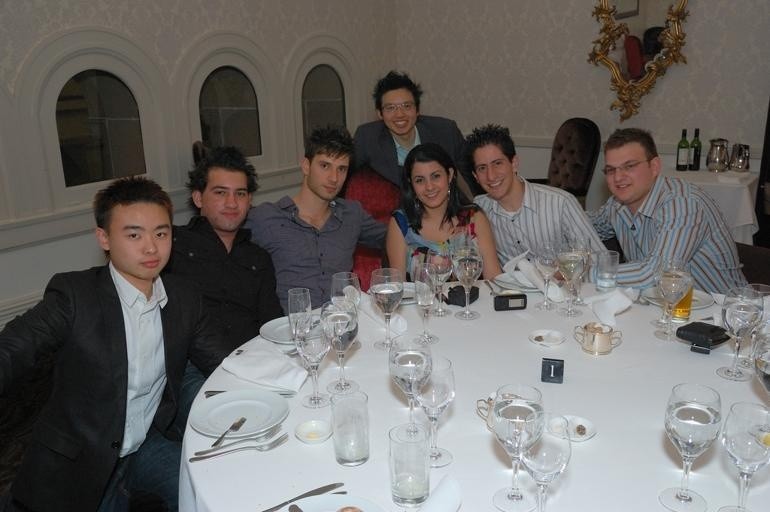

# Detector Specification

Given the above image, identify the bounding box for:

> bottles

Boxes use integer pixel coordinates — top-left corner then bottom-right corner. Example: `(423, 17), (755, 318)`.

(675, 127), (702, 170)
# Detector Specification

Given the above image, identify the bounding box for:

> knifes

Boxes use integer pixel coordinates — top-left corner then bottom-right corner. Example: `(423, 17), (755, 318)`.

(205, 388), (297, 397)
(262, 482), (344, 512)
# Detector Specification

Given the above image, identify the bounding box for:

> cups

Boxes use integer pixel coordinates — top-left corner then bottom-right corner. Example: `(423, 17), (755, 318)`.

(671, 287), (692, 323)
(330, 391), (371, 467)
(388, 421), (431, 510)
(595, 249), (620, 292)
(574, 322), (623, 355)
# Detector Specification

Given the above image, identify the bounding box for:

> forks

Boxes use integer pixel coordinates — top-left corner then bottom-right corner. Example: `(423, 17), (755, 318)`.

(189, 417), (288, 463)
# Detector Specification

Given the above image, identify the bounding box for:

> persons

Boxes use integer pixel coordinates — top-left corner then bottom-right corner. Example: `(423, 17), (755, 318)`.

(351, 71), (487, 202)
(160, 145), (286, 442)
(384, 142), (504, 282)
(464, 124), (612, 274)
(240, 124), (388, 315)
(553, 128), (754, 298)
(0, 172), (235, 512)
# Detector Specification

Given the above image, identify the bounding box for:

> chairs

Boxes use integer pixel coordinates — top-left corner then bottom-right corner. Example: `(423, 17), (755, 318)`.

(524, 116), (602, 212)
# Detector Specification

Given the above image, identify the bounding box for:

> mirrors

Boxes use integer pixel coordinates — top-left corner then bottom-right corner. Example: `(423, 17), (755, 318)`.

(586, 0), (690, 123)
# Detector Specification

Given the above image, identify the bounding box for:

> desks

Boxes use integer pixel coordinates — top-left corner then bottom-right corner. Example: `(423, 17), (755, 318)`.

(662, 164), (757, 249)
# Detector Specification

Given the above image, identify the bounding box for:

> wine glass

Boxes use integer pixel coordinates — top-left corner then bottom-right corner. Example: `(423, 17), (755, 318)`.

(489, 383), (572, 510)
(716, 283), (770, 383)
(413, 264), (439, 346)
(387, 334), (433, 445)
(371, 268), (404, 355)
(534, 237), (591, 320)
(296, 316), (331, 408)
(287, 288), (312, 337)
(332, 272), (362, 352)
(424, 247), (453, 319)
(410, 357), (456, 468)
(650, 255), (691, 329)
(450, 248), (483, 320)
(657, 381), (769, 512)
(321, 299), (360, 395)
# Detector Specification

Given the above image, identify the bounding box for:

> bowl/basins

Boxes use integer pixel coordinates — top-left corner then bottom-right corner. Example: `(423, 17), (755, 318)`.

(295, 420), (332, 444)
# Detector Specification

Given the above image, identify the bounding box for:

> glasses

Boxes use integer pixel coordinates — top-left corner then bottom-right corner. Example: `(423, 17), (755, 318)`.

(605, 160), (647, 174)
(381, 103), (414, 112)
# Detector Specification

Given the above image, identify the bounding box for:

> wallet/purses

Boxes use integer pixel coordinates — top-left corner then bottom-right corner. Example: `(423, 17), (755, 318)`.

(676, 321), (731, 346)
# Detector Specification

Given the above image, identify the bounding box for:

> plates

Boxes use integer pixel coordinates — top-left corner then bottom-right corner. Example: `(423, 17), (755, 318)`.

(549, 415), (597, 441)
(494, 270), (560, 292)
(259, 315), (326, 344)
(367, 282), (435, 305)
(529, 330), (565, 346)
(641, 286), (715, 310)
(189, 391), (290, 437)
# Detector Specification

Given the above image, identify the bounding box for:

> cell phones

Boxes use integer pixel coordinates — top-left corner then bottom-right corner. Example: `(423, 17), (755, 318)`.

(494, 295), (528, 310)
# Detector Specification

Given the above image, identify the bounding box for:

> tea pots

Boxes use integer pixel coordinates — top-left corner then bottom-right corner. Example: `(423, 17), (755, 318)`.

(706, 137), (751, 173)
